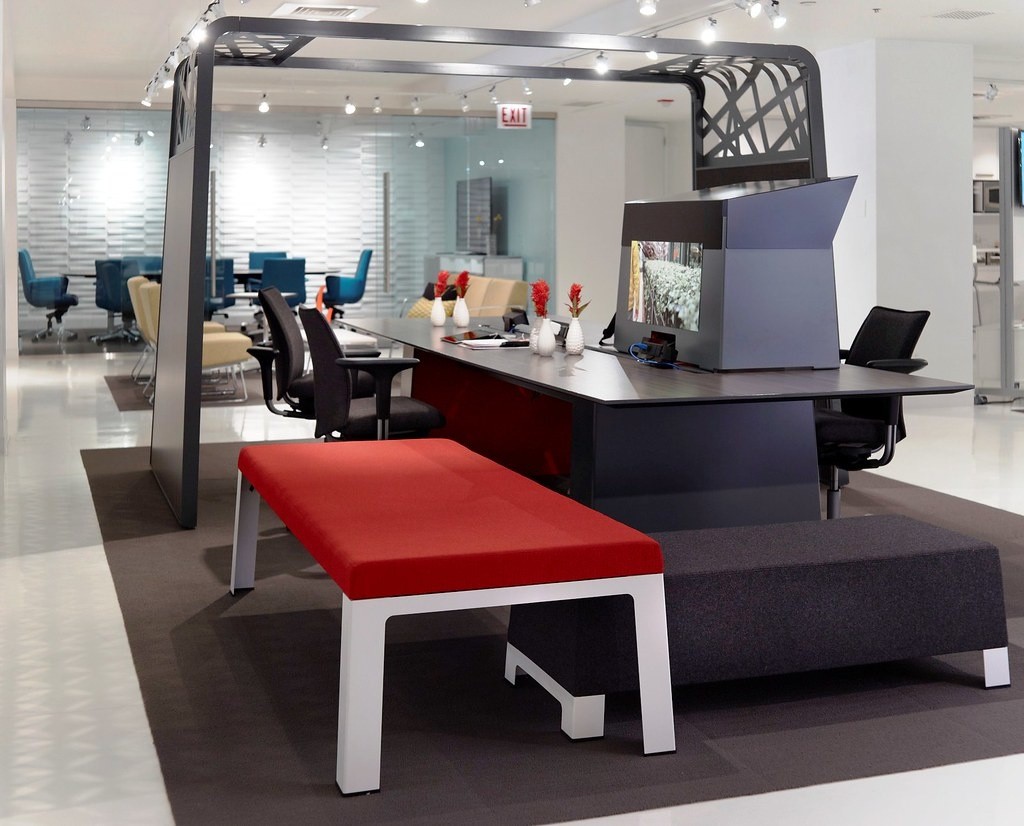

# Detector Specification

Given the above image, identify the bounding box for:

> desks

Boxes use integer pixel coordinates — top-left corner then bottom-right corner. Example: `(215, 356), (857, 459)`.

(334, 317), (977, 528)
(59, 268), (340, 285)
(225, 290), (299, 343)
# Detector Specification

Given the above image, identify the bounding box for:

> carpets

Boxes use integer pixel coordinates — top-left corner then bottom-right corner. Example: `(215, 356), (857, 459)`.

(15, 329), (312, 354)
(103, 369), (285, 412)
(78, 445), (1024, 826)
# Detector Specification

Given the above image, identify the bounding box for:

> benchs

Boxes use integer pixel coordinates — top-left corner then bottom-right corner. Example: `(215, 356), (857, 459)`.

(389, 273), (529, 359)
(231, 438), (1012, 797)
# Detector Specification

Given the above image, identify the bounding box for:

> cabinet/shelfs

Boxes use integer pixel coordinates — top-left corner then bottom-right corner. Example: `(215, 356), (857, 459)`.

(436, 251), (524, 278)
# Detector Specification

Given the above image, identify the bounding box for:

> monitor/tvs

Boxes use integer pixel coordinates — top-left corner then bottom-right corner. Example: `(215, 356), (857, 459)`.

(454, 178), (492, 255)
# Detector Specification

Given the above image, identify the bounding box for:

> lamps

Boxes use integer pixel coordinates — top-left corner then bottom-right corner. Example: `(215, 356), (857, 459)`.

(461, 0), (786, 112)
(344, 97), (382, 113)
(410, 96), (424, 147)
(160, 66), (174, 89)
(258, 94), (269, 114)
(141, 85), (157, 108)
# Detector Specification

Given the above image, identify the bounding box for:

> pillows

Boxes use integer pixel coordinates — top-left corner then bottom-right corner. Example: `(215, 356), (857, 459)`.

(423, 282), (457, 301)
(406, 295), (456, 318)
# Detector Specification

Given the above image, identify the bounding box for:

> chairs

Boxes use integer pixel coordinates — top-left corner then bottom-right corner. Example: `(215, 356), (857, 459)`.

(18, 248), (80, 342)
(88, 248), (444, 443)
(815, 307), (931, 523)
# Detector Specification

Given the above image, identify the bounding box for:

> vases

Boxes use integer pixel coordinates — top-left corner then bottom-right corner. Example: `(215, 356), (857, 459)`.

(487, 235), (498, 256)
(430, 296), (470, 327)
(528, 317), (584, 357)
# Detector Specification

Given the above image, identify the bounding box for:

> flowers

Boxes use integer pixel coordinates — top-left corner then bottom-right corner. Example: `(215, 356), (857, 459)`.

(474, 214), (502, 233)
(434, 270), (470, 298)
(529, 279), (591, 317)
